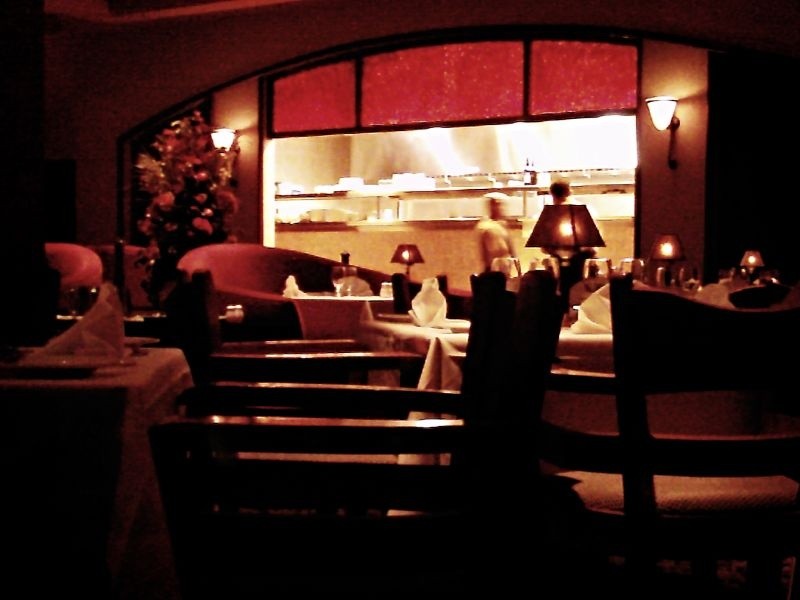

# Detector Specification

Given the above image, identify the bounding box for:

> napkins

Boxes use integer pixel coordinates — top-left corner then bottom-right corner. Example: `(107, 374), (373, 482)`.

(41, 281), (132, 363)
(569, 288), (611, 336)
(404, 276), (454, 329)
(330, 274), (376, 299)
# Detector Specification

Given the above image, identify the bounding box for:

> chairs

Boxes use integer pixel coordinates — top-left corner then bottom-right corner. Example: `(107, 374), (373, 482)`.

(149, 266), (800, 600)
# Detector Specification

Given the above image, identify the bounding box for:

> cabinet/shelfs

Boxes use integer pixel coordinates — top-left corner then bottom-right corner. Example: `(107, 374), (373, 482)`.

(270, 172), (640, 234)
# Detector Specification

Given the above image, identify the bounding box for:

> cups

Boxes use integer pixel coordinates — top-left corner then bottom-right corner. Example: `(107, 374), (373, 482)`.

(730, 267), (778, 290)
(656, 266), (700, 293)
(582, 257), (645, 296)
(380, 282), (393, 297)
(491, 255), (562, 295)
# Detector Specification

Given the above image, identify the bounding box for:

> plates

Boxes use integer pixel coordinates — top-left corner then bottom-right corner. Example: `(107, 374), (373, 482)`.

(123, 336), (162, 347)
(4, 355), (137, 376)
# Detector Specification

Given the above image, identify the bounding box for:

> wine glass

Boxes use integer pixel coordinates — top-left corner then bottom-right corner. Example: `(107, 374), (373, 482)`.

(330, 265), (358, 296)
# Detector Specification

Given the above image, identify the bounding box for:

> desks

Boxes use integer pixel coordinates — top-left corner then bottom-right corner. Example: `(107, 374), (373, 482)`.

(360, 313), (612, 465)
(0, 345), (198, 579)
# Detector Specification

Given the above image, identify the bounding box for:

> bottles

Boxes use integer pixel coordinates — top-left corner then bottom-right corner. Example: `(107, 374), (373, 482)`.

(332, 252), (358, 277)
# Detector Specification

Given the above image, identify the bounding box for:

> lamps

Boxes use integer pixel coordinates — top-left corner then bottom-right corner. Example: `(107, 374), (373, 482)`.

(522, 201), (610, 285)
(646, 227), (687, 284)
(645, 93), (683, 134)
(209, 125), (242, 155)
(388, 242), (427, 278)
(738, 250), (766, 282)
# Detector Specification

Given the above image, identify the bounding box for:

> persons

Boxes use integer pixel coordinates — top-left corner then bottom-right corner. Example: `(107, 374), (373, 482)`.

(475, 195), (522, 278)
(541, 181), (595, 315)
